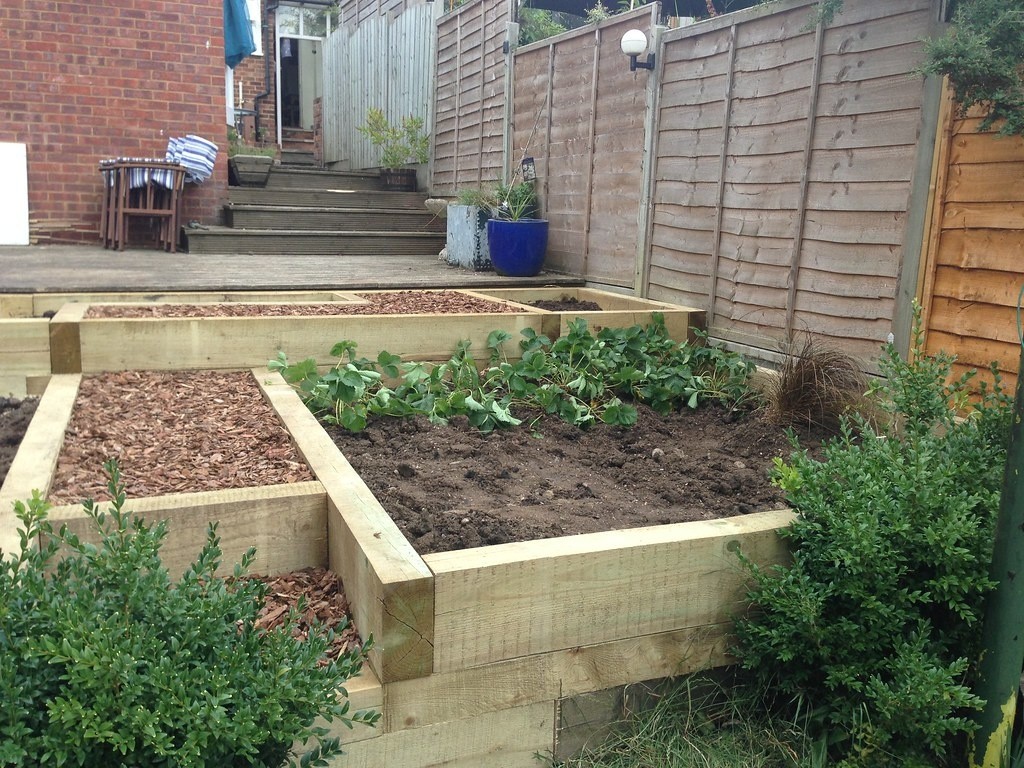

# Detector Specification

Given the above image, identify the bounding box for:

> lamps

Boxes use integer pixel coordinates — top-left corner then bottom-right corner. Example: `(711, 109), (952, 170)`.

(620, 29), (655, 71)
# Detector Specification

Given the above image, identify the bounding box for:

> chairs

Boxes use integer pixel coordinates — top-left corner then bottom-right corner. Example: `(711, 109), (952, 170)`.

(97, 134), (219, 254)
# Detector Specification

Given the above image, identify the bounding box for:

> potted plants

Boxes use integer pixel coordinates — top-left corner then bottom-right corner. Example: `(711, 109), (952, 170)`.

(228, 145), (275, 188)
(356, 107), (432, 192)
(447, 168), (550, 277)
